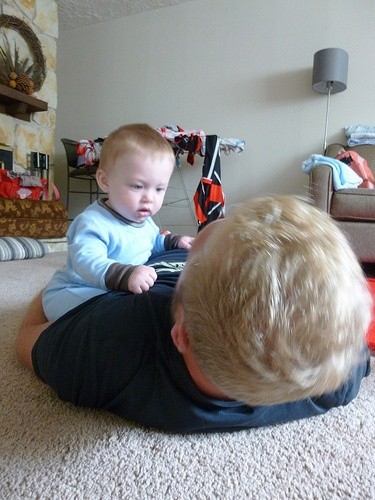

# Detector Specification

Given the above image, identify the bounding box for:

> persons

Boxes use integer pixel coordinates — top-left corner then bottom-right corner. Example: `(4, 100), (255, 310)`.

(42, 123), (195, 322)
(15, 194), (371, 432)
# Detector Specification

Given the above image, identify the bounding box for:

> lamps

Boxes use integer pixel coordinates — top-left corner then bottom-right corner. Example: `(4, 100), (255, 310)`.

(312, 48), (348, 154)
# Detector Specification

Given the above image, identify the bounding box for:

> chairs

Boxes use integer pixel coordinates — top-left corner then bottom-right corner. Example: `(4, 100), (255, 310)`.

(61, 138), (98, 221)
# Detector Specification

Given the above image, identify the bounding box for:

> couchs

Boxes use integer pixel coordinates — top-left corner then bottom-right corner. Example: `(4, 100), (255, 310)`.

(311, 143), (375, 262)
